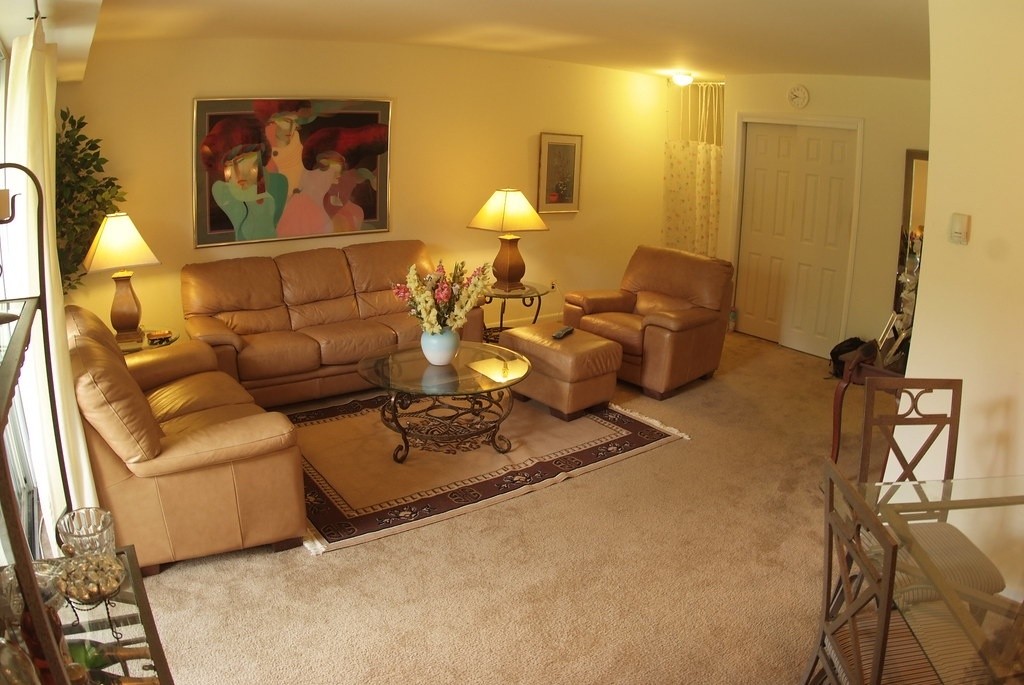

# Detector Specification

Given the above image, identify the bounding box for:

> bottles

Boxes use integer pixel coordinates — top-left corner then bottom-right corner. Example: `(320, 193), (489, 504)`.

(0, 566), (161, 685)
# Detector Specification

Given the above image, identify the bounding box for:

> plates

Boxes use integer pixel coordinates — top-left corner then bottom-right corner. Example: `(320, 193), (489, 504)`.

(52, 557), (126, 601)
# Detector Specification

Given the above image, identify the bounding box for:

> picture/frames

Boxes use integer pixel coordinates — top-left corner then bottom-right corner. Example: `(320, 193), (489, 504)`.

(189, 96), (394, 250)
(537, 132), (584, 215)
(878, 310), (897, 351)
(884, 331), (910, 367)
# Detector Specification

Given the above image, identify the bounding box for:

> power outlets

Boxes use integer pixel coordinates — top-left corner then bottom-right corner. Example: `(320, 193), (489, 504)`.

(550, 281), (557, 292)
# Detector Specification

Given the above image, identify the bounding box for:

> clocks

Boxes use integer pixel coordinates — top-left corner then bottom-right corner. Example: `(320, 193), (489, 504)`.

(788, 85), (808, 109)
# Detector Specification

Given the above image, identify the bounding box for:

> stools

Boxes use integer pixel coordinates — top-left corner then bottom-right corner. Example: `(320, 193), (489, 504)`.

(499, 321), (623, 423)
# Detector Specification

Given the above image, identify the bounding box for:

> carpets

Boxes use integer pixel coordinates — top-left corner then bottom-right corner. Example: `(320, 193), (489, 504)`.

(266, 366), (681, 557)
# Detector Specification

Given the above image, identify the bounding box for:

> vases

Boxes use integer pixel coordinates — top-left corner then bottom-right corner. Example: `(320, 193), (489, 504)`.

(420, 326), (459, 368)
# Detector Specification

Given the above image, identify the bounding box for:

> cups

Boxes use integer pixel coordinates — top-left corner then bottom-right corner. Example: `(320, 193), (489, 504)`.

(56, 507), (115, 556)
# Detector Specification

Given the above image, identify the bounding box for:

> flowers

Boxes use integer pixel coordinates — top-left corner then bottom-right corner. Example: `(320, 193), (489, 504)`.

(392, 258), (498, 331)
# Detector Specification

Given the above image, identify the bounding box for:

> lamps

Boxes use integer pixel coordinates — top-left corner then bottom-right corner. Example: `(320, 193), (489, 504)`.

(467, 189), (547, 290)
(84, 211), (162, 354)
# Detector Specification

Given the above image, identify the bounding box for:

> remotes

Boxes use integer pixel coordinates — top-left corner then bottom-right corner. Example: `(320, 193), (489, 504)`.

(552, 327), (574, 339)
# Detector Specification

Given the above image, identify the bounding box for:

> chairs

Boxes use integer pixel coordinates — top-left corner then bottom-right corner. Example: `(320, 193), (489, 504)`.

(821, 376), (1006, 632)
(798, 458), (995, 683)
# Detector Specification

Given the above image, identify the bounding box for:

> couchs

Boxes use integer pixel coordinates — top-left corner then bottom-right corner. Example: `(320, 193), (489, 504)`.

(181, 237), (483, 411)
(57, 303), (308, 569)
(563, 242), (737, 396)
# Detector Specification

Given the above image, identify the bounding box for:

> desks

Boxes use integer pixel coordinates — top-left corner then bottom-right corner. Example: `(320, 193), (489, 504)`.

(831, 335), (908, 481)
(819, 475), (1024, 684)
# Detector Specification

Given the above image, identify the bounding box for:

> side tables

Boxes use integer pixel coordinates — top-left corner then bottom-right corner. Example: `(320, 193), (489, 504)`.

(468, 282), (551, 346)
(115, 327), (183, 357)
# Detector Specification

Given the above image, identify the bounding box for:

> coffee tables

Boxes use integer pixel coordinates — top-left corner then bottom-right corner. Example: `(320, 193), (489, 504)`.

(355, 342), (533, 463)
